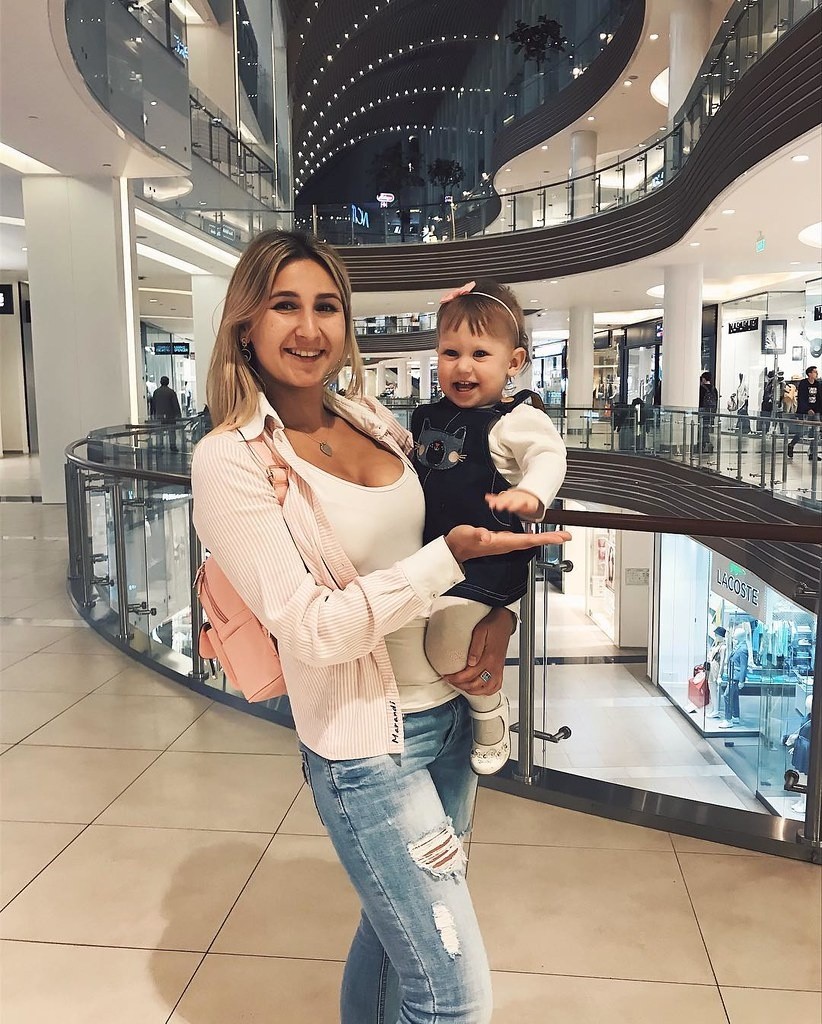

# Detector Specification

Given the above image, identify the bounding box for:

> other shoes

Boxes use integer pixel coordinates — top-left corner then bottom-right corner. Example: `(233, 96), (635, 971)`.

(467, 692), (511, 777)
(787, 442), (793, 457)
(171, 447), (179, 453)
(807, 453), (820, 461)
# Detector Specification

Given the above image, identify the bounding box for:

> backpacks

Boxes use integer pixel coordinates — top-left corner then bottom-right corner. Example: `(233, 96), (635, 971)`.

(191, 418), (336, 704)
(737, 400), (752, 433)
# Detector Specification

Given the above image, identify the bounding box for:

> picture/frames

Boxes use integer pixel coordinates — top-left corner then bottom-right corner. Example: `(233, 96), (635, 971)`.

(792, 346), (803, 361)
(761, 320), (787, 354)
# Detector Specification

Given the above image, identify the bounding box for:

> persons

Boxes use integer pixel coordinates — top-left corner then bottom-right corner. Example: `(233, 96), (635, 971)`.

(718, 628), (748, 728)
(410, 279), (566, 776)
(151, 376), (181, 453)
(706, 626), (728, 717)
(761, 371), (786, 432)
(785, 695), (813, 812)
(734, 374), (749, 433)
(699, 373), (718, 453)
(788, 366), (822, 461)
(192, 227), (572, 1024)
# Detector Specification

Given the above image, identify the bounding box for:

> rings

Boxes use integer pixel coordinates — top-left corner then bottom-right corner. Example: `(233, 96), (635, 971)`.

(480, 670), (491, 682)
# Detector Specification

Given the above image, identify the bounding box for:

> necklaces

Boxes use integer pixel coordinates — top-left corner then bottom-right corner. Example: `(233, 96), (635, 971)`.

(280, 410), (332, 457)
